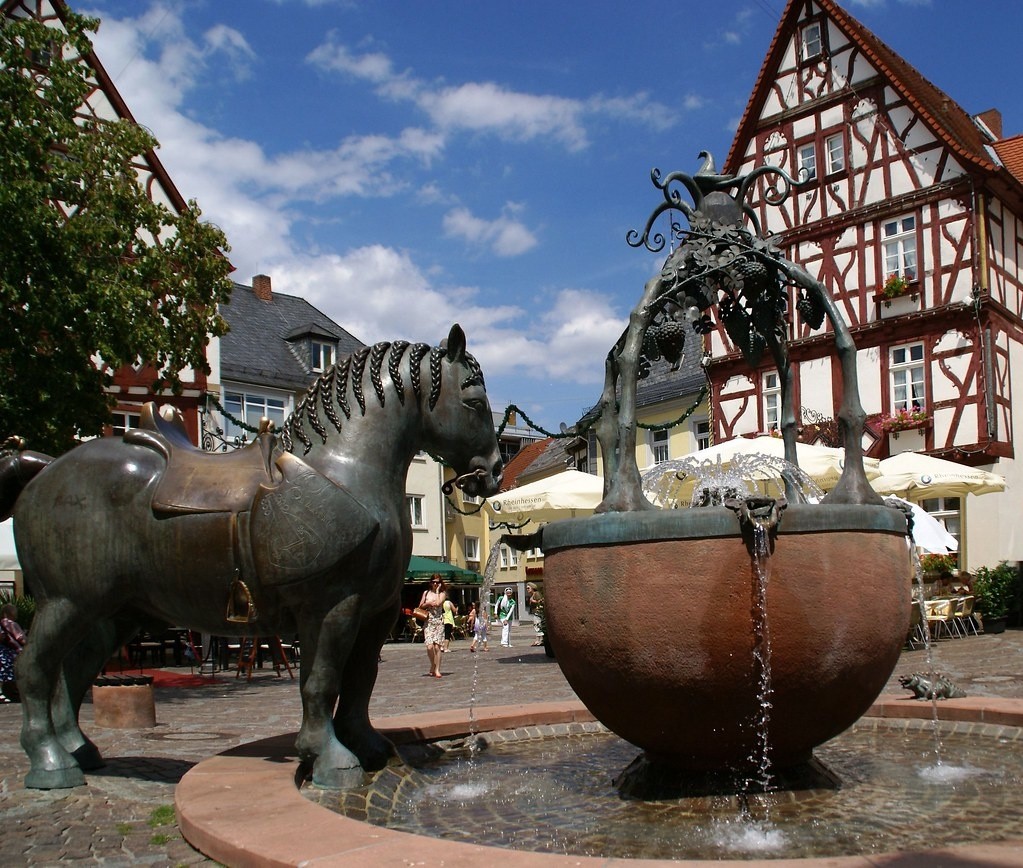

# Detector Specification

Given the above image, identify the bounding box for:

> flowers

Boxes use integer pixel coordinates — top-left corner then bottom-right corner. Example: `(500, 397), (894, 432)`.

(769, 429), (784, 439)
(920, 552), (958, 575)
(878, 273), (914, 297)
(874, 406), (933, 432)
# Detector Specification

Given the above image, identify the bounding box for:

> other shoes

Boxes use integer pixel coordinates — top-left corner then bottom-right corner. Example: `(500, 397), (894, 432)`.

(976, 629), (986, 634)
(444, 649), (451, 652)
(471, 645), (476, 652)
(484, 648), (488, 651)
(502, 644), (508, 648)
(532, 641), (545, 646)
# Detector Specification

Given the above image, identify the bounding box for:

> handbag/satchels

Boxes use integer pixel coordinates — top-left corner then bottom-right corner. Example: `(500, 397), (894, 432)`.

(413, 607), (430, 621)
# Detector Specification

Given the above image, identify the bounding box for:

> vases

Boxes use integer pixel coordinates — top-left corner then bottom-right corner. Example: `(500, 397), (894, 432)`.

(926, 568), (942, 577)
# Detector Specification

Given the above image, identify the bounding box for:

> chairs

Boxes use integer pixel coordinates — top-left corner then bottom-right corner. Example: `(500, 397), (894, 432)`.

(906, 595), (979, 650)
(99, 604), (477, 675)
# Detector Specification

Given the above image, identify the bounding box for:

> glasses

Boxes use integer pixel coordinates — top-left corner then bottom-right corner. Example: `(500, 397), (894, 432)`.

(506, 589), (512, 591)
(526, 587), (530, 589)
(431, 581), (439, 584)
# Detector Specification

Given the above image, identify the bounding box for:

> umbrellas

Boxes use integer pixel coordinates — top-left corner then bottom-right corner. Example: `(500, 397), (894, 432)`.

(635, 432), (751, 498)
(822, 447), (1007, 506)
(480, 463), (672, 525)
(687, 429), (887, 509)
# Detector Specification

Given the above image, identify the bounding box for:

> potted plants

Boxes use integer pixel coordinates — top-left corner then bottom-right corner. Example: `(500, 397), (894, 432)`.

(967, 559), (1019, 634)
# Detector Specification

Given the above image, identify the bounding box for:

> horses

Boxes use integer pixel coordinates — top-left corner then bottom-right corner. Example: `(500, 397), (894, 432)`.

(2, 323), (504, 790)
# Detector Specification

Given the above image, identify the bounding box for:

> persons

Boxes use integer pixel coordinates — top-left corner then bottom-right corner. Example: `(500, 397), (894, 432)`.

(442, 591), (458, 653)
(494, 586), (516, 647)
(0, 602), (29, 704)
(959, 572), (985, 634)
(416, 571), (445, 680)
(526, 581), (544, 646)
(407, 612), (424, 640)
(471, 597), (489, 653)
(929, 570), (959, 598)
(461, 602), (476, 633)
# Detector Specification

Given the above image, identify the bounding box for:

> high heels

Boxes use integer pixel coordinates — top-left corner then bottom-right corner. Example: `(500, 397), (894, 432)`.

(429, 664), (436, 675)
(435, 669), (442, 677)
